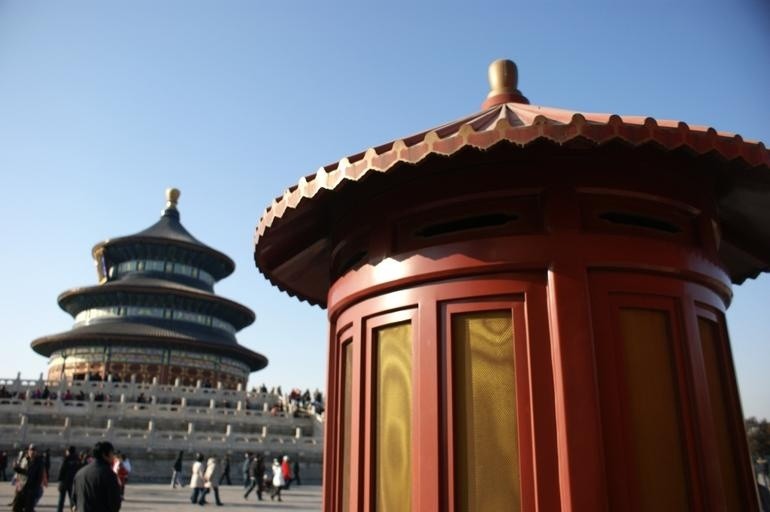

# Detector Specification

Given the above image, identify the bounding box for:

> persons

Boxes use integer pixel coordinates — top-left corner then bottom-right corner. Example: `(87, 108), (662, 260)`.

(137, 392), (146, 408)
(171, 400), (178, 411)
(0, 372), (120, 408)
(204, 379), (211, 393)
(250, 384), (322, 419)
(245, 401), (250, 415)
(754, 456), (770, 512)
(224, 400), (231, 414)
